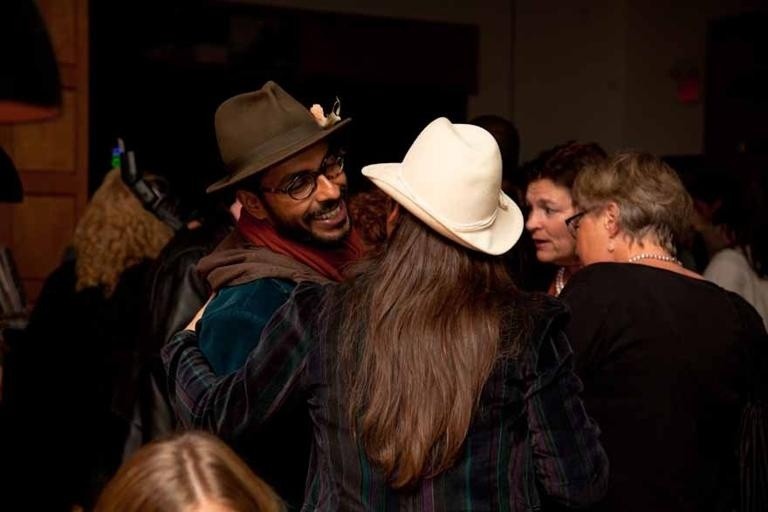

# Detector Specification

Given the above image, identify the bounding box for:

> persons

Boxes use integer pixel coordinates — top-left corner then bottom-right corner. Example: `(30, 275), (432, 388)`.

(161, 117), (768, 512)
(1, 82), (347, 511)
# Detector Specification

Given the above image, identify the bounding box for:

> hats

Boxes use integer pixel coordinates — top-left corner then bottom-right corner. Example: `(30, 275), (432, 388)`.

(206, 80), (351, 194)
(361, 116), (524, 257)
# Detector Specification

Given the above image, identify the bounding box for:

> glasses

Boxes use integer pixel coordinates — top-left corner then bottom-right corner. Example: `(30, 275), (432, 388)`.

(564, 206), (597, 240)
(255, 149), (345, 200)
